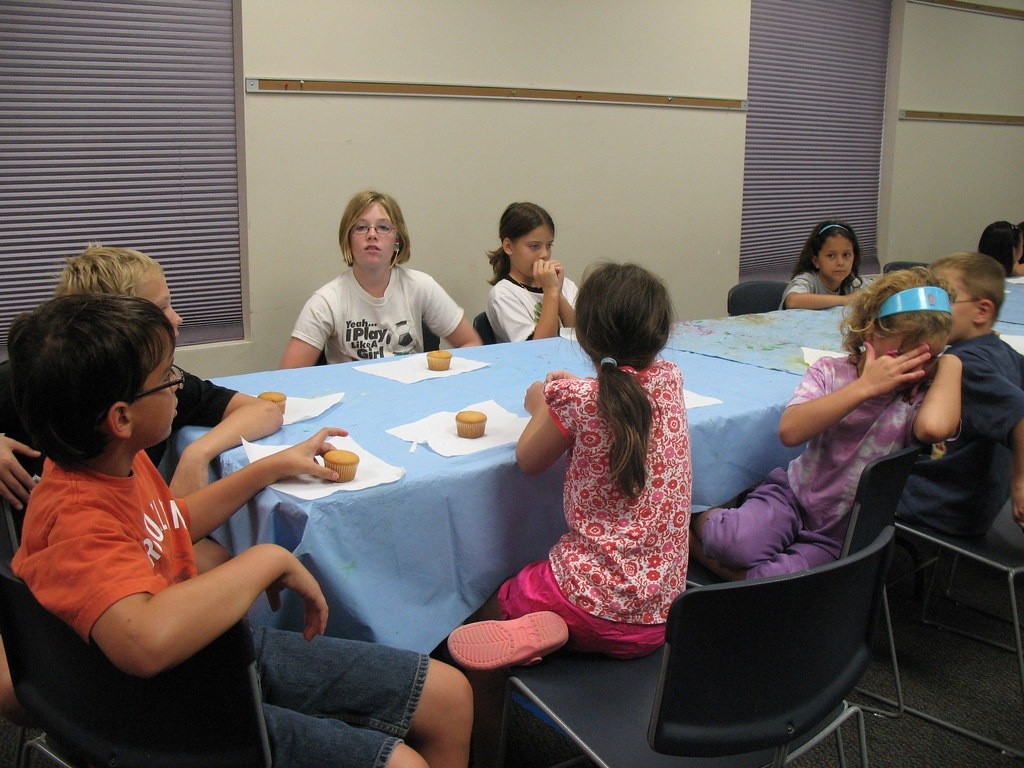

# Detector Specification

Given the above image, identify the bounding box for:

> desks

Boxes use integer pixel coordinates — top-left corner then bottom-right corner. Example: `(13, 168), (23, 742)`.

(153, 278), (1024, 676)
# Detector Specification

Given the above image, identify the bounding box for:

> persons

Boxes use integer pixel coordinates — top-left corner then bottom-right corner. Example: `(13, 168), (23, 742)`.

(280, 191), (483, 370)
(6, 291), (475, 768)
(486, 202), (580, 343)
(0, 241), (284, 729)
(779, 220), (875, 311)
(895, 252), (1024, 537)
(690, 266), (963, 582)
(447, 261), (693, 670)
(978, 220), (1024, 277)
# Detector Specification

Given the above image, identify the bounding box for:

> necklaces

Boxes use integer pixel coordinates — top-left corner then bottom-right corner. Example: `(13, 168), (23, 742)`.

(511, 273), (543, 305)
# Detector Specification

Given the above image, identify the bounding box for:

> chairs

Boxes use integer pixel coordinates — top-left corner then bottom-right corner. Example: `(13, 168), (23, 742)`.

(0, 534), (276, 768)
(688, 430), (941, 718)
(881, 259), (931, 274)
(855, 477), (1024, 758)
(0, 448), (38, 559)
(494, 523), (899, 768)
(725, 278), (789, 317)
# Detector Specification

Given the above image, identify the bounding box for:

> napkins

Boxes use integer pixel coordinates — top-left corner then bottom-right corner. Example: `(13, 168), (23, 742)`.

(348, 347), (493, 386)
(239, 427), (408, 502)
(557, 324), (579, 342)
(801, 347), (851, 368)
(1004, 276), (1024, 285)
(382, 398), (529, 460)
(247, 388), (347, 426)
(683, 381), (726, 413)
(999, 334), (1024, 355)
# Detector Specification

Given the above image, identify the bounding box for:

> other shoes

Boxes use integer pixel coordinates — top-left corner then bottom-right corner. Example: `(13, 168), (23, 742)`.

(447, 611), (569, 672)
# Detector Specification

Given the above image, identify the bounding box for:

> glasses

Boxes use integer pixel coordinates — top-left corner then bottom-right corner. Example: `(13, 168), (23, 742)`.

(127, 366), (186, 399)
(352, 224), (395, 235)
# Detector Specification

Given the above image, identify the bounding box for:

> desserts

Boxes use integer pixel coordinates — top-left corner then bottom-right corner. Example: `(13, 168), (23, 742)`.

(257, 392), (287, 415)
(427, 350), (452, 371)
(323, 449), (360, 482)
(456, 411), (487, 439)
